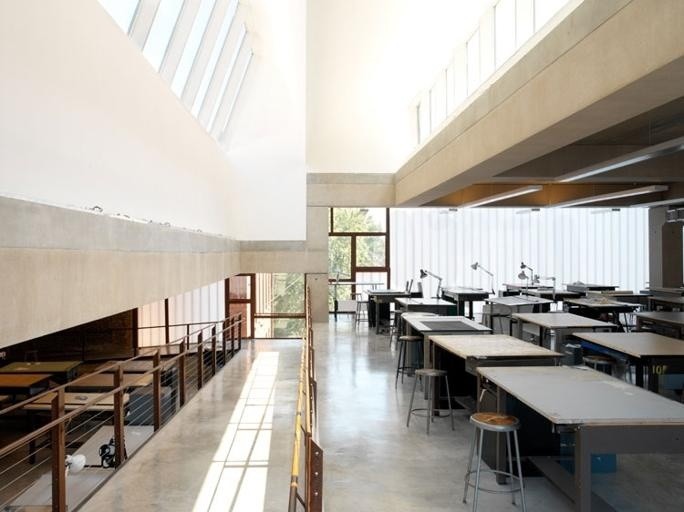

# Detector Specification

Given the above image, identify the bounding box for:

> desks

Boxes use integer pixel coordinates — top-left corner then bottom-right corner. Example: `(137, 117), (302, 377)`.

(354, 282), (684, 512)
(0, 340), (240, 511)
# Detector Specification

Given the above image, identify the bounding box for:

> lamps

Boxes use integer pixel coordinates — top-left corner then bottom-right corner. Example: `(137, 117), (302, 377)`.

(455, 116), (684, 214)
(419, 260), (557, 303)
